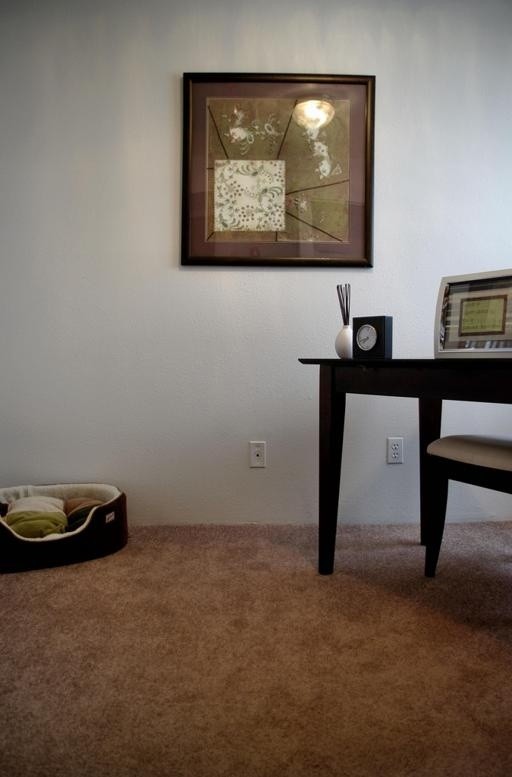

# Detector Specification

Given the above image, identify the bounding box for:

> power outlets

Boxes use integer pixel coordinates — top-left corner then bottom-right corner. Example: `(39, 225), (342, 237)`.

(386, 436), (404, 464)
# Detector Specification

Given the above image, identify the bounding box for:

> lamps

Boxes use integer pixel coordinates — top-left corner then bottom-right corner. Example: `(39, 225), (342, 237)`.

(291, 93), (335, 132)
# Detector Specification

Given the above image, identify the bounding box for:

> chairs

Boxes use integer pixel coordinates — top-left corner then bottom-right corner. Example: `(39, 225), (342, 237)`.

(424, 431), (512, 578)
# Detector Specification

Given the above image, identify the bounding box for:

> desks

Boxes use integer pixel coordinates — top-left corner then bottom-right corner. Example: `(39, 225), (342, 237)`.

(295, 356), (512, 577)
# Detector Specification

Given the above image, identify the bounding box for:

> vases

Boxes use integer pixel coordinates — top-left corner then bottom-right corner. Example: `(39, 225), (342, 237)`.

(335, 326), (353, 359)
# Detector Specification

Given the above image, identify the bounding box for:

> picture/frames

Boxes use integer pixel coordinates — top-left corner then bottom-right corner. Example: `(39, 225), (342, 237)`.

(180, 70), (376, 270)
(433, 268), (512, 358)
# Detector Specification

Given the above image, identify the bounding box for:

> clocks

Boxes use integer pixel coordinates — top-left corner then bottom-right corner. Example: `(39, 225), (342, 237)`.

(351, 316), (394, 358)
